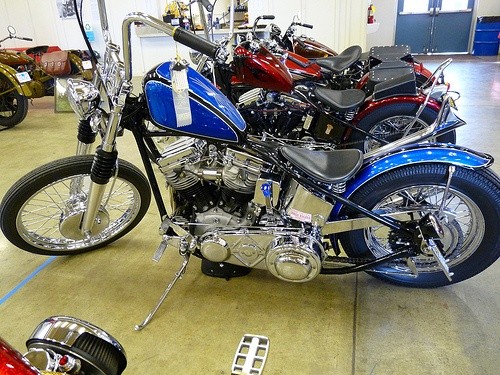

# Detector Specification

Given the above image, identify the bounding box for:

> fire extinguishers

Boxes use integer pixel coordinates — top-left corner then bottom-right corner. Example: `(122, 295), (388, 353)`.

(367, 3), (375, 24)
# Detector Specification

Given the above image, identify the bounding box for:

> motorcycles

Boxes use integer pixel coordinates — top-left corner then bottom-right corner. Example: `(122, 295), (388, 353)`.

(0, 0), (500, 338)
(160, 0), (467, 202)
(0, 25), (105, 129)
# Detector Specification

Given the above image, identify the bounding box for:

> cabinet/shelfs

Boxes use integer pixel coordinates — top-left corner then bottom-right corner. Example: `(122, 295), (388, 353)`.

(139, 27), (266, 72)
